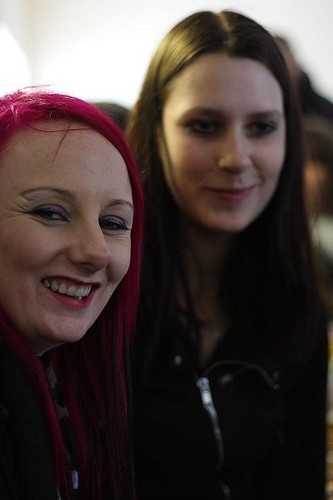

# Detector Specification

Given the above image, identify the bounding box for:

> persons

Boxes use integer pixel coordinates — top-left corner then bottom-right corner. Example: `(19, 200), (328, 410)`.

(0, 9), (332, 500)
